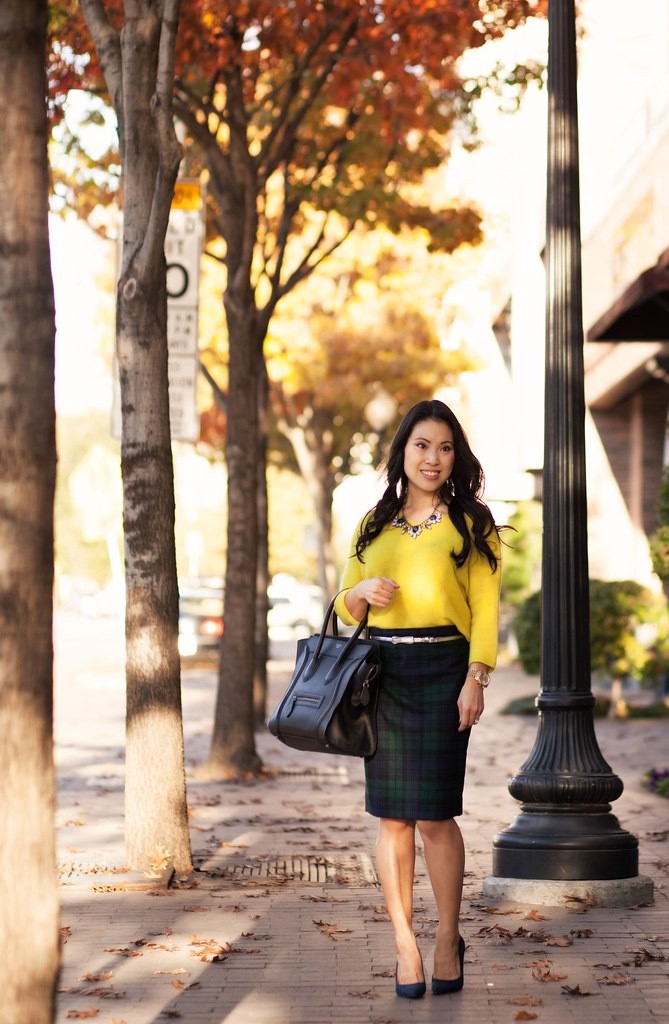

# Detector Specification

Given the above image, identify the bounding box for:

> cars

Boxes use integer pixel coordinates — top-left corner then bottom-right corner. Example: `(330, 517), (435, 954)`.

(264, 570), (327, 665)
(180, 589), (226, 665)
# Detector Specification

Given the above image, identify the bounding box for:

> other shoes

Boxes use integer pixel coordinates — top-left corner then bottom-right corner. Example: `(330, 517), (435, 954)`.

(395, 961), (426, 997)
(432, 934), (465, 993)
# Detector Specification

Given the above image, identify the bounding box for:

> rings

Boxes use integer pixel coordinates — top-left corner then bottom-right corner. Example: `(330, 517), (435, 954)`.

(474, 719), (479, 723)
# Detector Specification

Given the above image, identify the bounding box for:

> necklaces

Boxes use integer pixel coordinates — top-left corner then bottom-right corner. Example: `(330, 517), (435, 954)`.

(392, 497), (444, 538)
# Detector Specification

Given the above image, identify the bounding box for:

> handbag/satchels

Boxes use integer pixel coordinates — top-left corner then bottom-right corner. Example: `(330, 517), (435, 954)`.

(268, 588), (380, 758)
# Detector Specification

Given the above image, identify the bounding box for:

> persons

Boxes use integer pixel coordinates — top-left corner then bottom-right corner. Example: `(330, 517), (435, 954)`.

(331, 400), (502, 1000)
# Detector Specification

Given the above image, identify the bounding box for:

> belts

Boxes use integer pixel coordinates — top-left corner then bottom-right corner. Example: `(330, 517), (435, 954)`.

(371, 634), (463, 643)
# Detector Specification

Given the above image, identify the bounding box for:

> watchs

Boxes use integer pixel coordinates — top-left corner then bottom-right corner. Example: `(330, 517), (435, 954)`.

(465, 668), (491, 688)
